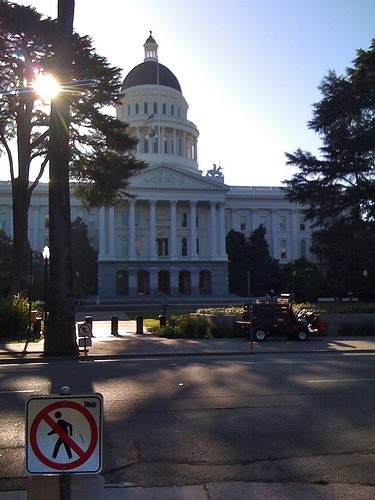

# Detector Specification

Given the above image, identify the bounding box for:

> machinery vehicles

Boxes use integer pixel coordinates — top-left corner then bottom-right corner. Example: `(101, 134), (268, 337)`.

(238, 293), (328, 342)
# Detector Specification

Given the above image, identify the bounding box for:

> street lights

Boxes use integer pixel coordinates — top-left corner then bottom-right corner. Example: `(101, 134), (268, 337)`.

(42, 245), (50, 321)
(363, 269), (369, 307)
